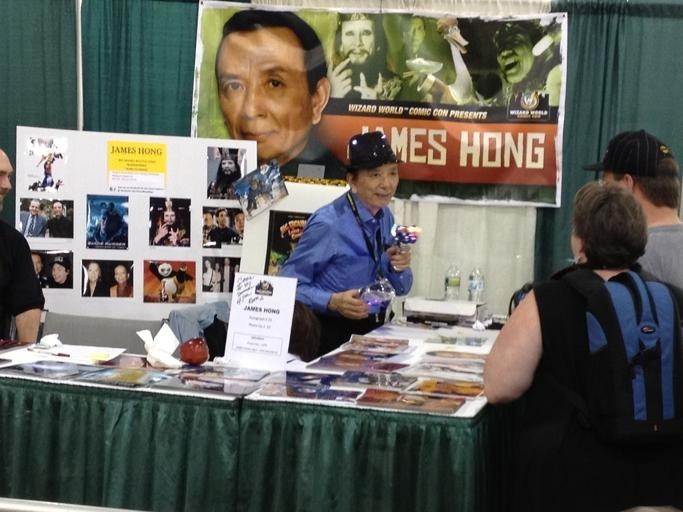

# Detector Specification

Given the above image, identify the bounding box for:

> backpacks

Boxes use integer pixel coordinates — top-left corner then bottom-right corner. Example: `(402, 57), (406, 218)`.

(563, 263), (683, 442)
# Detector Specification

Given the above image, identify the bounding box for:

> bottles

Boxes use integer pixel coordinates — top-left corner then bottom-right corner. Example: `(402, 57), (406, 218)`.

(467, 264), (485, 303)
(443, 261), (462, 302)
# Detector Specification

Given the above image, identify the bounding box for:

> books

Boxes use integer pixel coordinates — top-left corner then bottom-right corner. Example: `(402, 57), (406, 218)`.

(1, 330), (490, 416)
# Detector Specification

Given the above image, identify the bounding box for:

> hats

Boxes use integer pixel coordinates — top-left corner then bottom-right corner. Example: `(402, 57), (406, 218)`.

(582, 129), (681, 177)
(343, 132), (404, 171)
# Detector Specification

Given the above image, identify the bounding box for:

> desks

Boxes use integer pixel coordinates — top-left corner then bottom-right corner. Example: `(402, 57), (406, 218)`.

(240, 315), (505, 512)
(1, 337), (298, 512)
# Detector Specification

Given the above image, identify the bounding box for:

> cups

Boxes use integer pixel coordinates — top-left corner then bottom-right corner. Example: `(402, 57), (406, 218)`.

(362, 289), (380, 314)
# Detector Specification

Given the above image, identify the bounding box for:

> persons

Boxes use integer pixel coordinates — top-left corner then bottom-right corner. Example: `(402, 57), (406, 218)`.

(18, 145), (248, 300)
(270, 130), (413, 343)
(221, 10), (350, 182)
(577, 130), (683, 291)
(331, 11), (562, 112)
(481, 176), (682, 511)
(0, 149), (45, 347)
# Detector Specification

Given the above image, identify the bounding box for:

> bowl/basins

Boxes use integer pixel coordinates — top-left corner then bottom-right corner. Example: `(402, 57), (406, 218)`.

(179, 337), (210, 367)
